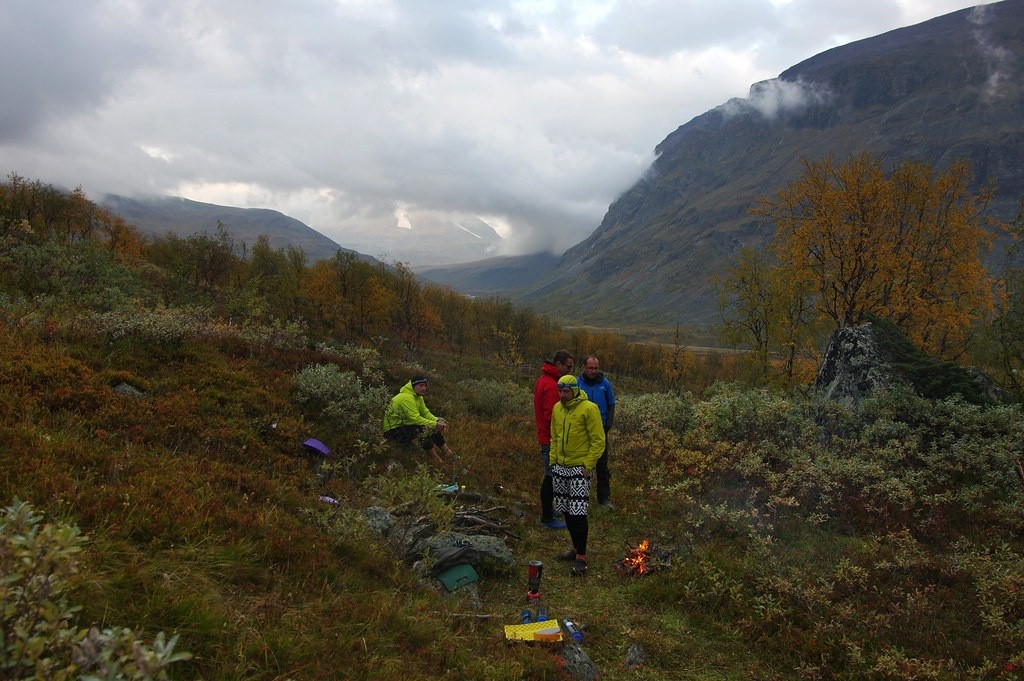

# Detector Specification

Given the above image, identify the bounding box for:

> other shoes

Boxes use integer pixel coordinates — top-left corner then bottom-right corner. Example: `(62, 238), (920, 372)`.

(570, 558), (588, 575)
(552, 511), (563, 517)
(543, 519), (567, 529)
(425, 455), (444, 466)
(555, 550), (576, 563)
(441, 448), (456, 457)
(599, 503), (615, 513)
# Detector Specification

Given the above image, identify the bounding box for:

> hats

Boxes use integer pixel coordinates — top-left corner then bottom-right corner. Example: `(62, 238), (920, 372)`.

(411, 375), (428, 388)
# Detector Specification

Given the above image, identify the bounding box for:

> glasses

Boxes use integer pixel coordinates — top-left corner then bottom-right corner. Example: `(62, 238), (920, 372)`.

(585, 366), (600, 371)
(559, 360), (572, 370)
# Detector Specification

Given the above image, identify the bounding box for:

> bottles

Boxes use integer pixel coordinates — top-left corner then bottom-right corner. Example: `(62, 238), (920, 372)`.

(522, 610), (532, 623)
(561, 619), (582, 641)
(536, 607), (548, 621)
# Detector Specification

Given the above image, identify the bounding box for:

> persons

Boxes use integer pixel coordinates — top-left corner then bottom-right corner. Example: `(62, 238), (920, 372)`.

(534, 350), (575, 529)
(549, 374), (606, 575)
(383, 375), (462, 463)
(576, 356), (615, 509)
